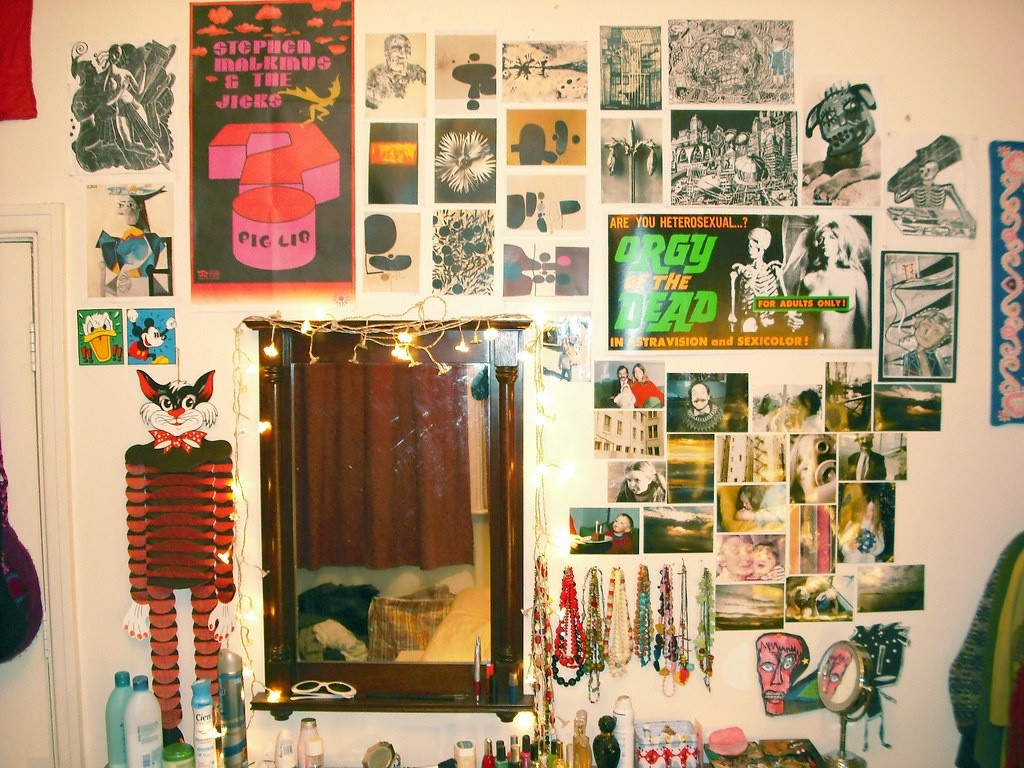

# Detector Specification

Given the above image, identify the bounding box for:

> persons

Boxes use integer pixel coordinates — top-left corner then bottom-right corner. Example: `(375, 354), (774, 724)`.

(733, 485), (786, 524)
(840, 494), (885, 563)
(676, 382), (724, 432)
(753, 389), (823, 434)
(714, 534), (785, 582)
(848, 432), (887, 481)
(795, 215), (870, 350)
(602, 362), (664, 408)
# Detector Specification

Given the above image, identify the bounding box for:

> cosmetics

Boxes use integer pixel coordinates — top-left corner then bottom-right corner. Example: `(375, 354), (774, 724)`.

(106, 651), (325, 768)
(362, 695), (636, 768)
(473, 636), (519, 705)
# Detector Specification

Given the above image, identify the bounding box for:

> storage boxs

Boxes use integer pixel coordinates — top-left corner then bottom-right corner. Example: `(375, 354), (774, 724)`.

(634, 721), (697, 768)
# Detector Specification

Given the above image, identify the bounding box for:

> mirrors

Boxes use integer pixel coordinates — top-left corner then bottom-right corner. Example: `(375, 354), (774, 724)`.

(361, 740), (402, 768)
(243, 314), (535, 723)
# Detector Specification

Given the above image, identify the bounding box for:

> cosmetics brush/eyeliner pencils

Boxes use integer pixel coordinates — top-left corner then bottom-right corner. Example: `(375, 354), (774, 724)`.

(366, 693), (467, 701)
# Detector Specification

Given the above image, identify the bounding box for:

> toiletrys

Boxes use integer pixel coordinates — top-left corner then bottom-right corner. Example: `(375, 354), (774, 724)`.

(274, 718), (324, 768)
(573, 695), (636, 768)
(105, 648), (248, 768)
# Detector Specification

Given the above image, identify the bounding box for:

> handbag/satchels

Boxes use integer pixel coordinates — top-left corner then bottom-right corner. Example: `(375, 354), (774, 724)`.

(366, 584), (456, 662)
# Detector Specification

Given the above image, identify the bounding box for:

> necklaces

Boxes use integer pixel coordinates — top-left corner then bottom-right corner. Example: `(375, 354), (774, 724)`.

(677, 564), (695, 687)
(693, 568), (716, 693)
(604, 568), (635, 678)
(580, 565), (606, 705)
(633, 564), (654, 666)
(651, 564), (678, 697)
(529, 560), (556, 747)
(551, 566), (586, 687)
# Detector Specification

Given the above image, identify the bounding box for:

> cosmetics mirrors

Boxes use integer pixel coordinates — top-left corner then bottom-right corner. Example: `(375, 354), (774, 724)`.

(816, 640), (873, 768)
